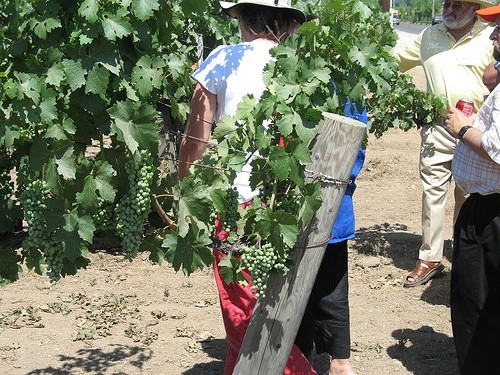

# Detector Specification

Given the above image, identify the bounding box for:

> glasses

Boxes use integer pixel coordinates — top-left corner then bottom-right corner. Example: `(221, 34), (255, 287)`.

(441, 1), (463, 10)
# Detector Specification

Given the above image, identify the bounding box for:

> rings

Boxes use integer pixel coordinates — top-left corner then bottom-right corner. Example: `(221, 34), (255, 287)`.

(447, 115), (451, 119)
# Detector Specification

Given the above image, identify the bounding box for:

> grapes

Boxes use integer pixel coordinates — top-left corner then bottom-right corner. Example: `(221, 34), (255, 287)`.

(0, 149), (290, 298)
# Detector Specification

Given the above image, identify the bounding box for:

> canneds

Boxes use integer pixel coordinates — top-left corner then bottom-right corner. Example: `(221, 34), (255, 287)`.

(455, 98), (474, 117)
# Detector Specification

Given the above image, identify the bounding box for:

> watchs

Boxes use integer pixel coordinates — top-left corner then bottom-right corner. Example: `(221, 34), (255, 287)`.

(493, 60), (500, 73)
(458, 125), (474, 145)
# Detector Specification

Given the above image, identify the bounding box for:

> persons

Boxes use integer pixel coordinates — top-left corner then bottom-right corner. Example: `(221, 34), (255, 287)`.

(445, 4), (500, 375)
(389, 0), (500, 288)
(176, 0), (368, 375)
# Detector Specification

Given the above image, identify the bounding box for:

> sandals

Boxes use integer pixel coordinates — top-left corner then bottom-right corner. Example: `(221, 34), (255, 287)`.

(404, 259), (444, 287)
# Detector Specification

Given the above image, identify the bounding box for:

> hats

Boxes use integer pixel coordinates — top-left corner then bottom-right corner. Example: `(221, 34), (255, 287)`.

(219, 0), (307, 25)
(458, 0), (494, 9)
(475, 4), (500, 22)
